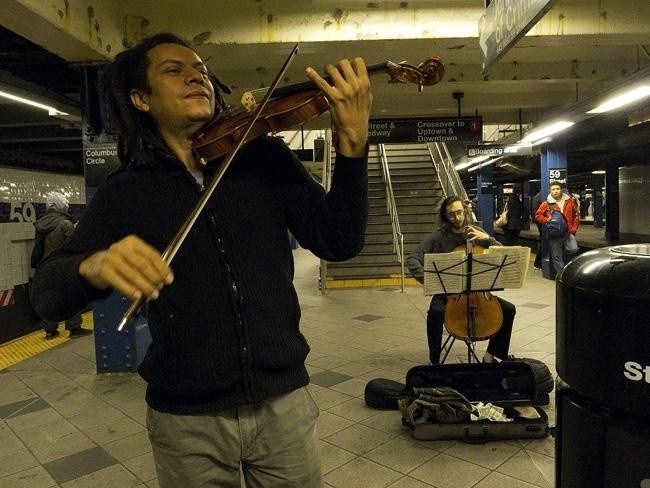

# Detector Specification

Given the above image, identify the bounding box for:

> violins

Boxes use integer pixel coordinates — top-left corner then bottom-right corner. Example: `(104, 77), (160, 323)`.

(191, 56), (446, 172)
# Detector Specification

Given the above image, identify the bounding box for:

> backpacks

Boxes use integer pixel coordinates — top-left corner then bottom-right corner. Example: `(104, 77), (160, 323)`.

(397, 385), (474, 425)
(544, 208), (570, 239)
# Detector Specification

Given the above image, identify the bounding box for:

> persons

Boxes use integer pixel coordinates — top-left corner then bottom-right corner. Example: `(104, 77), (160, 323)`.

(531, 191), (541, 270)
(499, 191), (530, 245)
(28, 33), (375, 487)
(535, 181), (580, 271)
(406, 196), (516, 366)
(571, 189), (590, 219)
(31, 191), (93, 338)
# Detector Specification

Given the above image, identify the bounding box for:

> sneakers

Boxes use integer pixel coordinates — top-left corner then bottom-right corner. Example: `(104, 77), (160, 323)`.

(43, 324), (94, 338)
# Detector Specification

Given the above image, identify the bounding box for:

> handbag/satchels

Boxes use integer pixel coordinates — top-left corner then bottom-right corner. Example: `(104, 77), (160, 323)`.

(494, 201), (511, 227)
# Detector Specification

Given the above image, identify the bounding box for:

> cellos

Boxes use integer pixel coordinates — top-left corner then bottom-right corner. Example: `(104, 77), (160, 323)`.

(443, 198), (504, 342)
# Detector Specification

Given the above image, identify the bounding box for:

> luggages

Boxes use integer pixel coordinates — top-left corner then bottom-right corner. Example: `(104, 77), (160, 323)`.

(401, 361), (550, 445)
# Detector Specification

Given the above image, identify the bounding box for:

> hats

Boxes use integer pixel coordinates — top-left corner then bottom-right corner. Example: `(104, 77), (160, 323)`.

(45, 191), (70, 215)
